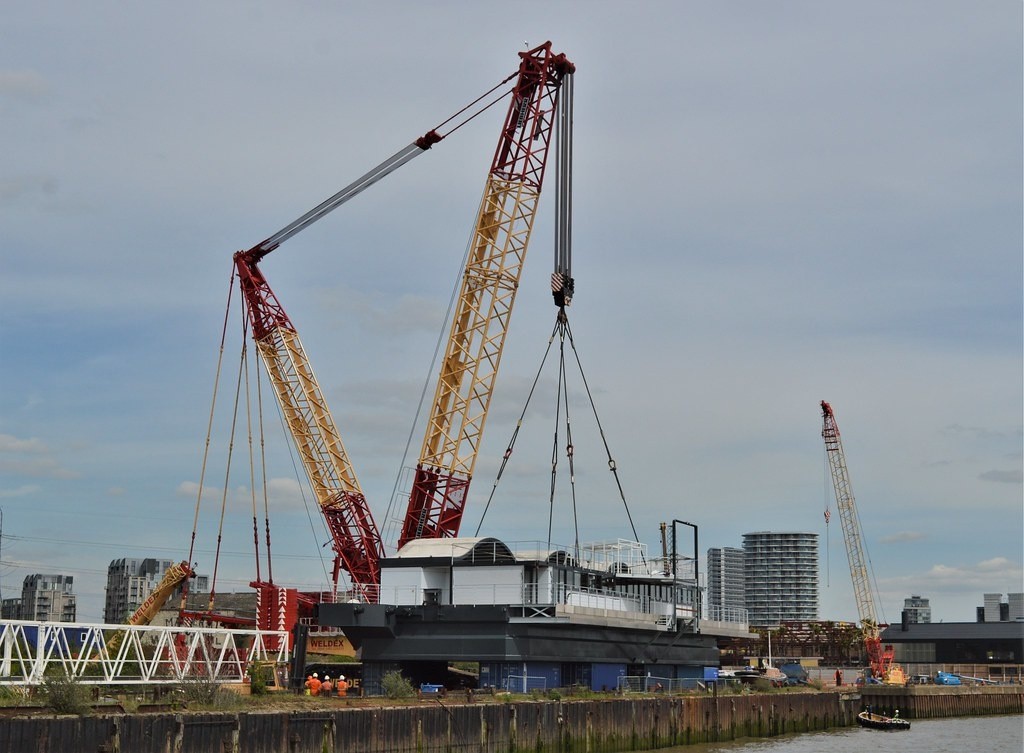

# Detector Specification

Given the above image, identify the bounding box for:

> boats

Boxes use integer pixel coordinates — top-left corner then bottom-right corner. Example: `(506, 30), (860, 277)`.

(858, 708), (910, 730)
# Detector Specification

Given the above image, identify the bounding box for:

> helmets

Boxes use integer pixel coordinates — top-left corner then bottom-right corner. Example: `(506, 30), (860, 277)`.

(313, 673), (319, 678)
(339, 675), (346, 681)
(325, 675), (330, 680)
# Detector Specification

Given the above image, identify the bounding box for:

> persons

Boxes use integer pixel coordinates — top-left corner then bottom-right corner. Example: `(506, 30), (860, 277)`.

(305, 673), (321, 696)
(866, 705), (871, 719)
(337, 675), (348, 696)
(464, 686), (472, 702)
(319, 676), (332, 697)
(834, 667), (842, 686)
(895, 710), (899, 717)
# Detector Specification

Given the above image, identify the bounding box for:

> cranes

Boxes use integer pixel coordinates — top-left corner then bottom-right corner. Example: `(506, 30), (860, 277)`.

(86, 41), (650, 677)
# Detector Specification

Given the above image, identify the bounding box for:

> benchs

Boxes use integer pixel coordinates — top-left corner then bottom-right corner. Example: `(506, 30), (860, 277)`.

(418, 688), (446, 699)
(29, 684), (99, 702)
(619, 682), (650, 692)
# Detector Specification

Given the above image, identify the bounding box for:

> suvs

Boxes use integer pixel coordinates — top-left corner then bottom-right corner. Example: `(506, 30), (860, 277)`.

(421, 685), (447, 700)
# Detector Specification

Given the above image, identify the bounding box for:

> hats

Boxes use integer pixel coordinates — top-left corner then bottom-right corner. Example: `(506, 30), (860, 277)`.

(836, 667), (839, 670)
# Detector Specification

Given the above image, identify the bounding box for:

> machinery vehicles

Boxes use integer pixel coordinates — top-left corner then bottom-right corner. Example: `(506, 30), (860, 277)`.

(219, 660), (291, 696)
(819, 401), (910, 686)
